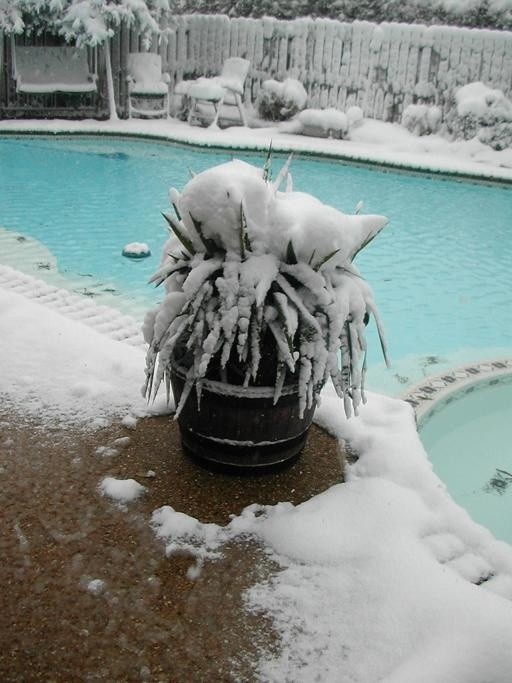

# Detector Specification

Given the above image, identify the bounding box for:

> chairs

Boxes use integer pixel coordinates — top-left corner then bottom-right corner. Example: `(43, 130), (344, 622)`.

(177, 58), (254, 129)
(124, 51), (170, 121)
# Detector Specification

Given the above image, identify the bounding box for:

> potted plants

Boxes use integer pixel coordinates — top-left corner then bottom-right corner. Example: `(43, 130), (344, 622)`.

(141, 141), (392, 480)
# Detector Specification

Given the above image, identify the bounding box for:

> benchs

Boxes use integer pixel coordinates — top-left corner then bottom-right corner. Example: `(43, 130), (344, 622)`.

(8, 32), (102, 94)
